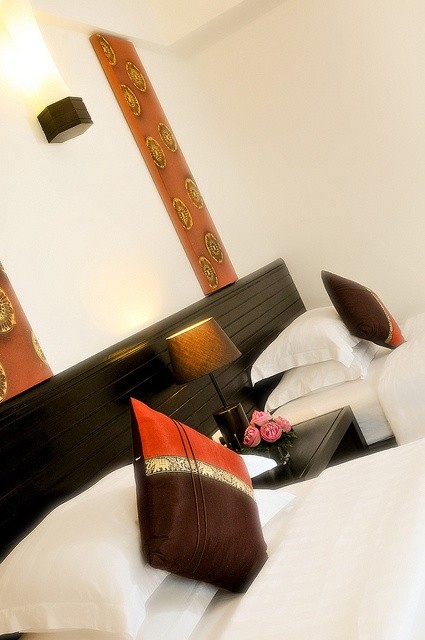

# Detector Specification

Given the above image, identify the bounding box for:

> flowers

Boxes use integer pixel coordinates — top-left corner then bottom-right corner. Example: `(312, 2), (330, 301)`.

(242, 410), (298, 450)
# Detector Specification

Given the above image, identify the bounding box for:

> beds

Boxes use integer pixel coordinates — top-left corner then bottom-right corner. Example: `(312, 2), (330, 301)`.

(0, 439), (425, 640)
(265, 319), (425, 444)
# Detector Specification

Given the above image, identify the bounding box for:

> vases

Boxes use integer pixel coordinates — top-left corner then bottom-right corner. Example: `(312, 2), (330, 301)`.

(266, 444), (291, 466)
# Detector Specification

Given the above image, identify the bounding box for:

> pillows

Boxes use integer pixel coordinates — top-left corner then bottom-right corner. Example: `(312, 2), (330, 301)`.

(319, 270), (406, 350)
(0, 452), (279, 640)
(249, 307), (362, 388)
(128, 397), (269, 594)
(264, 342), (379, 412)
(18, 488), (295, 640)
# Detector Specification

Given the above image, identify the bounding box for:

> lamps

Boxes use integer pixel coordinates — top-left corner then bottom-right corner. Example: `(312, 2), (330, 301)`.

(36, 95), (94, 145)
(164, 317), (250, 453)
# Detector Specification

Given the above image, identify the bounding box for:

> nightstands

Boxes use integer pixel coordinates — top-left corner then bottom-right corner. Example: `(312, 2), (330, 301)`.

(280, 405), (368, 480)
